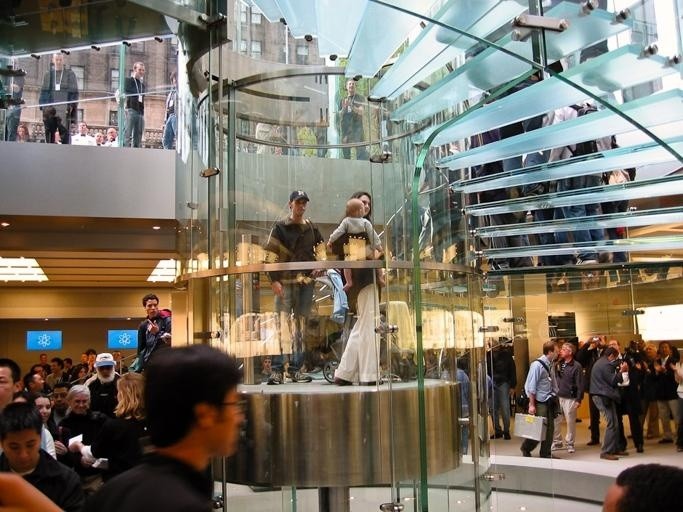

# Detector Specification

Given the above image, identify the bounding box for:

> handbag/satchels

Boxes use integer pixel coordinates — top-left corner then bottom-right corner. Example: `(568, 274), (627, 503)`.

(516, 391), (529, 413)
(547, 396), (561, 419)
(604, 169), (630, 184)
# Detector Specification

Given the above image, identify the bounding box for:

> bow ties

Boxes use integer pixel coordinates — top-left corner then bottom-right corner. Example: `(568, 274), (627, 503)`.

(98, 138), (115, 146)
(81, 134), (85, 136)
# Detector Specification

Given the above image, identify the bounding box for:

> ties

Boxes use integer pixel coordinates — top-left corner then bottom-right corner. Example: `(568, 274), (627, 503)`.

(615, 358), (623, 384)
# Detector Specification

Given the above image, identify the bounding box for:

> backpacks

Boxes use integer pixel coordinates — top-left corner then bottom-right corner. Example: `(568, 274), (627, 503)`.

(569, 104), (597, 157)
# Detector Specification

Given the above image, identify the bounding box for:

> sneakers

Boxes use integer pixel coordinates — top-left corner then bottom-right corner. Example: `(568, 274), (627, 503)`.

(520, 444), (532, 457)
(677, 446), (683, 452)
(603, 450), (628, 460)
(551, 443), (563, 451)
(566, 444), (576, 453)
(504, 433), (511, 440)
(647, 434), (673, 443)
(637, 448), (643, 452)
(545, 454), (554, 458)
(490, 433), (503, 439)
(587, 441), (599, 445)
(576, 259), (598, 266)
(268, 371), (283, 384)
(286, 372), (312, 383)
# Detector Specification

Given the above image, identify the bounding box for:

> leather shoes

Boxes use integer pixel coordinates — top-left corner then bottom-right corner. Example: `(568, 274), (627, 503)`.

(359, 380), (383, 385)
(334, 377), (351, 385)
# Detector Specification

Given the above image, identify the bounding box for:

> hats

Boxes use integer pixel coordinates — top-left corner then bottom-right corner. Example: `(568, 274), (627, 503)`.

(290, 190), (310, 202)
(96, 353), (116, 367)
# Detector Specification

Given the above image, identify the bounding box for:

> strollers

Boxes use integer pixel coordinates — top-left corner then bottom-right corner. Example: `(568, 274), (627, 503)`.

(300, 269), (412, 382)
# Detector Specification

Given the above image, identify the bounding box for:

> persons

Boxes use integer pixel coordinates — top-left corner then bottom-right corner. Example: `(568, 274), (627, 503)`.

(256, 336), (683, 460)
(39, 52), (79, 145)
(602, 463), (682, 511)
(162, 68), (177, 150)
(0, 57), (25, 141)
(332, 191), (387, 385)
(370, 3), (635, 282)
(1, 275), (246, 511)
(16, 122), (120, 148)
(338, 78), (369, 160)
(114, 62), (145, 147)
(326, 198), (386, 289)
(241, 121), (326, 157)
(264, 189), (325, 383)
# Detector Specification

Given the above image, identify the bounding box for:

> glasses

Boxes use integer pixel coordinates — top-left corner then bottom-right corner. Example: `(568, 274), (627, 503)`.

(205, 400), (250, 414)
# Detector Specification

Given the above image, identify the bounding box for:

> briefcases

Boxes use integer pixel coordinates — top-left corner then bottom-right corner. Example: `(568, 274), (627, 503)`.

(513, 413), (548, 441)
